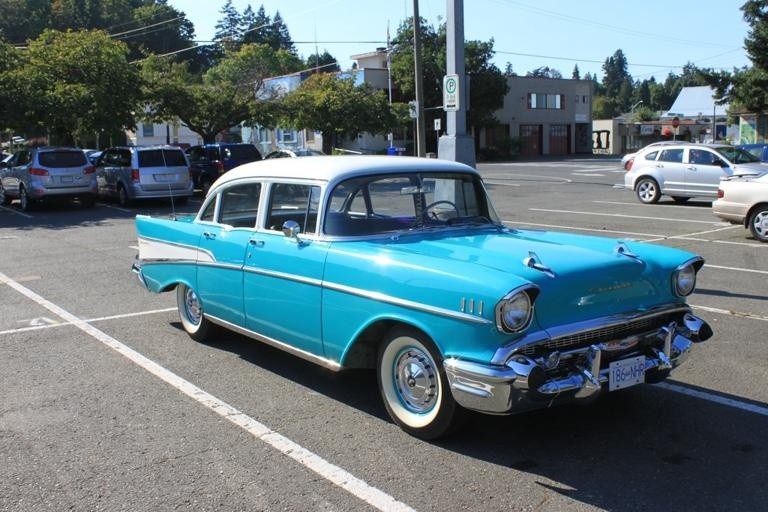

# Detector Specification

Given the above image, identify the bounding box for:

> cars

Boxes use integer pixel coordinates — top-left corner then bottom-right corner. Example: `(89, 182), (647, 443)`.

(262, 149), (326, 159)
(92, 145), (195, 207)
(622, 142), (768, 243)
(0, 149), (98, 211)
(184, 143), (261, 198)
(134, 157), (712, 441)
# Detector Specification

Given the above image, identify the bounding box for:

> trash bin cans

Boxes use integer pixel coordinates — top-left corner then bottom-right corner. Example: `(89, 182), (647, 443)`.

(387, 145), (408, 155)
